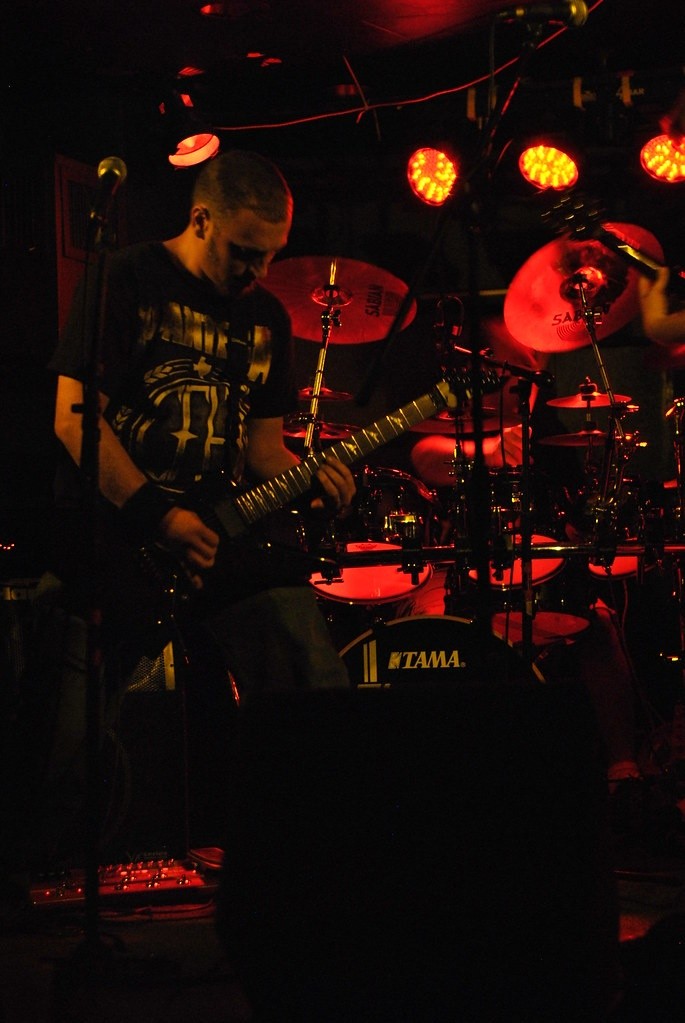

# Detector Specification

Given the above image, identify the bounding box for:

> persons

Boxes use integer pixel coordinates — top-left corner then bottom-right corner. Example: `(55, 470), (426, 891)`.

(2, 150), (362, 936)
(637, 264), (685, 350)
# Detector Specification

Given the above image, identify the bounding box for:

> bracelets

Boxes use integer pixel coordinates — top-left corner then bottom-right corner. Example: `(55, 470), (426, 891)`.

(118, 481), (174, 536)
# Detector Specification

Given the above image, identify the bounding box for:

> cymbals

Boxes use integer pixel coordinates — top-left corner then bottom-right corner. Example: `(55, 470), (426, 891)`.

(296, 387), (353, 401)
(538, 430), (609, 447)
(504, 222), (665, 352)
(258, 255), (416, 343)
(546, 393), (633, 408)
(281, 420), (362, 440)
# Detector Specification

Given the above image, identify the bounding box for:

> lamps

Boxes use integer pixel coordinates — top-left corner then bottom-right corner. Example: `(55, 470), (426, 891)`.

(153, 83), (222, 169)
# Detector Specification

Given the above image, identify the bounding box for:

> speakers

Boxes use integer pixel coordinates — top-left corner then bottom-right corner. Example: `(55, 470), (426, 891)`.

(214, 684), (619, 1023)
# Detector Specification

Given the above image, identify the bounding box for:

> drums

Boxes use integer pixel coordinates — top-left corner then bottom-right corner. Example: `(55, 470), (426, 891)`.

(338, 615), (546, 685)
(467, 532), (564, 613)
(311, 542), (435, 652)
(589, 539), (683, 660)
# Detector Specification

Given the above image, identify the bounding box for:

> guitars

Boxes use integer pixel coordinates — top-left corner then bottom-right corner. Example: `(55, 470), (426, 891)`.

(94, 364), (502, 646)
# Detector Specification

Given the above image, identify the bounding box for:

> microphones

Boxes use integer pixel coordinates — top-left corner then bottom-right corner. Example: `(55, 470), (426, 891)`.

(497, 0), (588, 29)
(87, 156), (128, 234)
